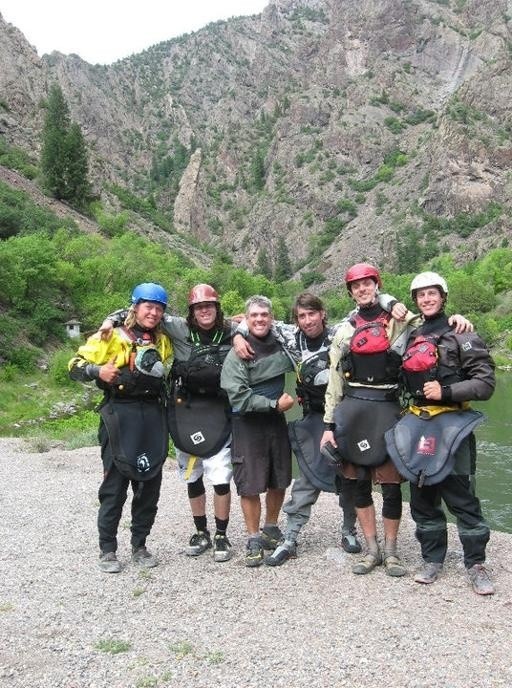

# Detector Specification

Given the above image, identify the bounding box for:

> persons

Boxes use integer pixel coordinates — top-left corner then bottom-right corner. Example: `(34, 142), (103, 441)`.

(320, 262), (474, 575)
(221, 296), (294, 566)
(67, 282), (243, 573)
(395, 269), (496, 595)
(99, 282), (243, 563)
(231, 294), (409, 566)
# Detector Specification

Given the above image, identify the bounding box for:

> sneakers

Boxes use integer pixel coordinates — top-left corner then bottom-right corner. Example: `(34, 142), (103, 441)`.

(259, 526), (289, 550)
(352, 547), (383, 575)
(414, 559), (445, 585)
(185, 529), (213, 557)
(97, 551), (122, 574)
(465, 563), (496, 597)
(132, 545), (159, 569)
(242, 534), (264, 568)
(265, 540), (297, 567)
(381, 550), (408, 577)
(212, 531), (232, 562)
(340, 531), (362, 554)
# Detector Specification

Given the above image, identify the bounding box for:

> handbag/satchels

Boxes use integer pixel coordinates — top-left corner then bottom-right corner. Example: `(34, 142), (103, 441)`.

(348, 320), (439, 374)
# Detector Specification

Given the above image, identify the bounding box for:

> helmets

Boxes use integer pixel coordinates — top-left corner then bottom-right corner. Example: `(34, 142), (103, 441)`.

(345, 263), (383, 290)
(130, 280), (170, 305)
(187, 282), (220, 307)
(411, 270), (450, 300)
